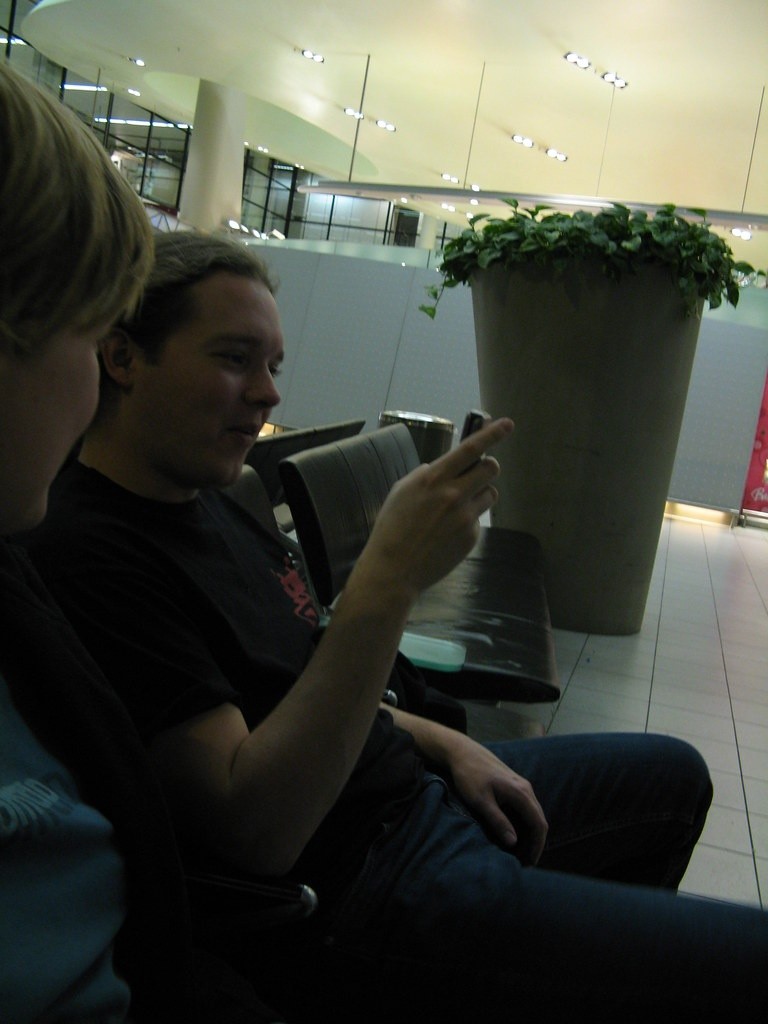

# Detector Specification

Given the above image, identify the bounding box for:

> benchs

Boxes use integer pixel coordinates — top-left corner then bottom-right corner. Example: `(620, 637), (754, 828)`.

(221, 418), (563, 733)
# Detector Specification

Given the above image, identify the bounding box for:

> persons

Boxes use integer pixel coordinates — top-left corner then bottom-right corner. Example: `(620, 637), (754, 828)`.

(1, 53), (767, 1024)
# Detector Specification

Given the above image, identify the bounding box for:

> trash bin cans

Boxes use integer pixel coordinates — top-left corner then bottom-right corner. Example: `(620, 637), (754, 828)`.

(379, 409), (454, 465)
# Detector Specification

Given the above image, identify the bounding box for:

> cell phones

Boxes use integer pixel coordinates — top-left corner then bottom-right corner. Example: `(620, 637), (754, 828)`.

(457, 408), (492, 475)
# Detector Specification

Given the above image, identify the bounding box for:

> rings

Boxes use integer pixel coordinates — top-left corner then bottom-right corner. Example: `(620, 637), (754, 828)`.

(488, 484), (499, 502)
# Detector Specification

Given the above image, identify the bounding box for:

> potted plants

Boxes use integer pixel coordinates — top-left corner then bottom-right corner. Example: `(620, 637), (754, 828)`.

(418, 197), (767, 638)
